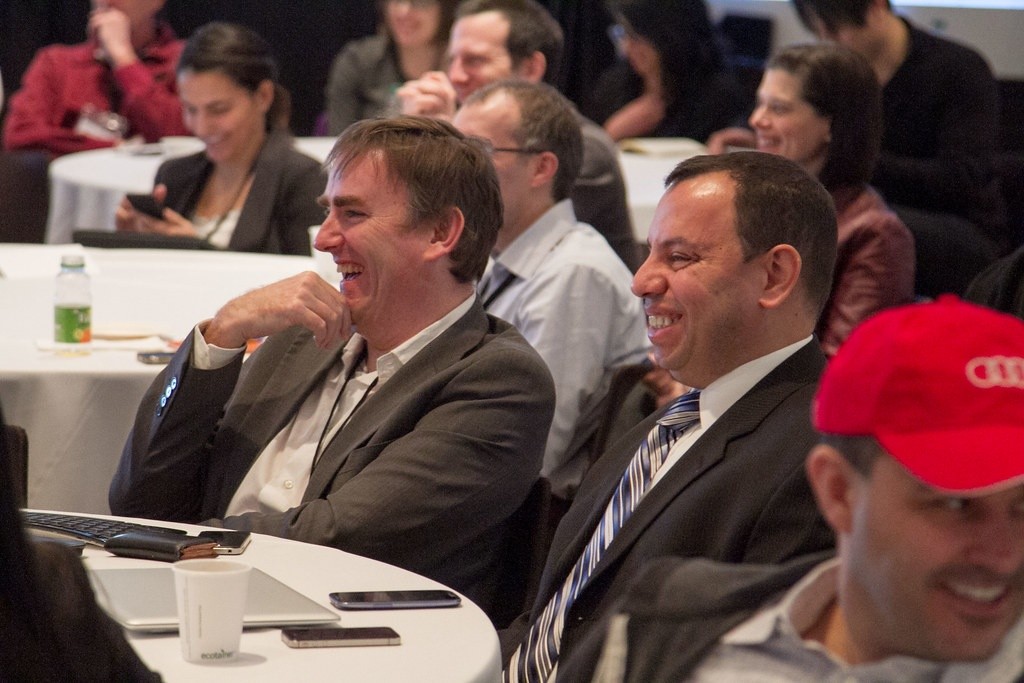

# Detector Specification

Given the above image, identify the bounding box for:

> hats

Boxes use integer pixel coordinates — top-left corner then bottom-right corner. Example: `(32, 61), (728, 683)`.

(811, 294), (1023, 490)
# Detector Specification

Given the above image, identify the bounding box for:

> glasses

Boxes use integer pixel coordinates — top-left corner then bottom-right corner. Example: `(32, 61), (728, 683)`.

(469, 136), (538, 157)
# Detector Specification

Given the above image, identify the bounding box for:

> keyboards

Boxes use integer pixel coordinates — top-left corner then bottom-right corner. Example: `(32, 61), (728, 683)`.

(19, 510), (188, 546)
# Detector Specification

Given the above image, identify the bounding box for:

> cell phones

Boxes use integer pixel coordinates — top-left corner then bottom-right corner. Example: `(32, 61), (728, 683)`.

(137, 352), (175, 364)
(281, 627), (400, 648)
(329, 589), (462, 610)
(126, 193), (165, 221)
(196, 530), (251, 556)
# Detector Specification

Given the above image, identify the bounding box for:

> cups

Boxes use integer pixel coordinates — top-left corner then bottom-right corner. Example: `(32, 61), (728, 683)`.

(172, 557), (251, 665)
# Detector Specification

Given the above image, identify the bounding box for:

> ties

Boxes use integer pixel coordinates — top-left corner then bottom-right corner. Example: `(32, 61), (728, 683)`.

(503, 392), (702, 683)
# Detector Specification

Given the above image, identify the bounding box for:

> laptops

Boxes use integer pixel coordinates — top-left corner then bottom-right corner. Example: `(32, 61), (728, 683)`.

(91, 566), (341, 631)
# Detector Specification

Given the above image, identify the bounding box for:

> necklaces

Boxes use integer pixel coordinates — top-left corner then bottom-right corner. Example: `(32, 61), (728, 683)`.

(204, 167), (251, 241)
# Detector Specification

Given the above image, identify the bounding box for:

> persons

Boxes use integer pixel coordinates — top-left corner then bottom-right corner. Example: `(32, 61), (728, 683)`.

(750, 44), (914, 363)
(107, 114), (556, 616)
(3, 0), (196, 156)
(691, 294), (1024, 683)
(114, 22), (330, 256)
(457, 83), (658, 532)
(499, 151), (838, 683)
(786, 0), (1010, 228)
(322, 1), (767, 276)
(0, 401), (164, 682)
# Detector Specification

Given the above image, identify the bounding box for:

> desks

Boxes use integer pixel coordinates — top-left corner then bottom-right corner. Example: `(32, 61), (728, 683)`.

(0, 241), (344, 514)
(21, 507), (503, 683)
(44, 135), (708, 265)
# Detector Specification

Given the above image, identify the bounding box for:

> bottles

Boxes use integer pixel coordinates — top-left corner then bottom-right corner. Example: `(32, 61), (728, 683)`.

(53, 253), (92, 356)
